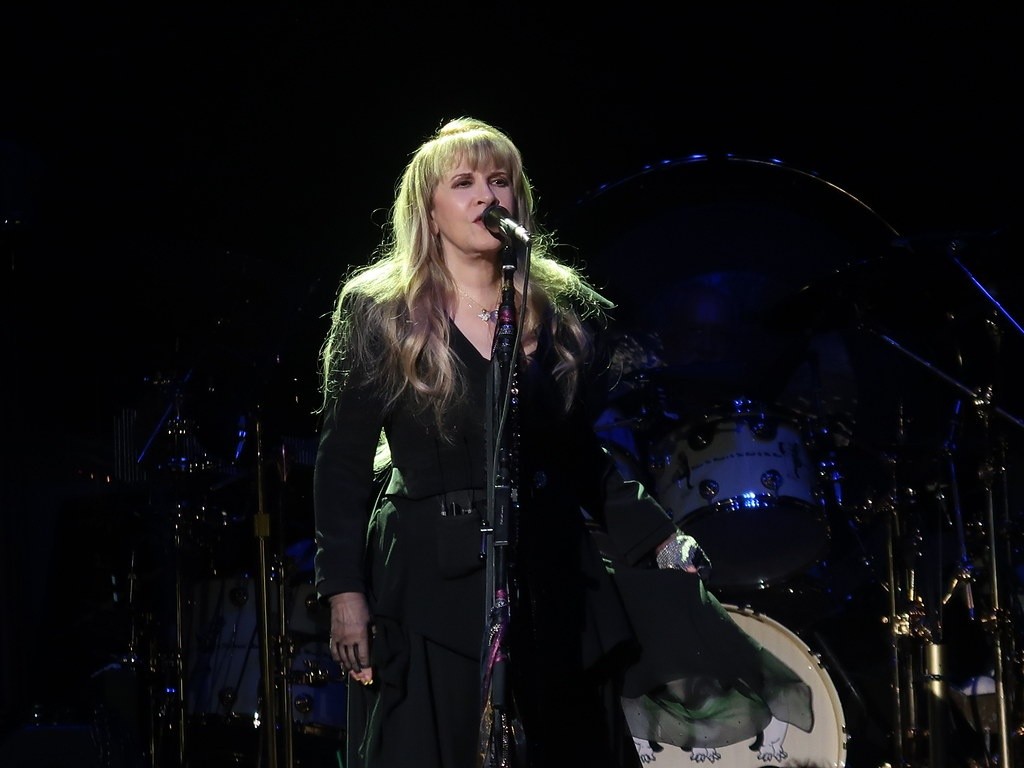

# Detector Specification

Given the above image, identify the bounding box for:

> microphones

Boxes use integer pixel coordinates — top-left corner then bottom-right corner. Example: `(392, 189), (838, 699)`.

(483, 205), (532, 246)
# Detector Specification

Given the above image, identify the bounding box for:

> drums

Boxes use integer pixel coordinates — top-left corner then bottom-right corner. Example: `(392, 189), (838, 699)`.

(618, 600), (855, 767)
(641, 404), (833, 593)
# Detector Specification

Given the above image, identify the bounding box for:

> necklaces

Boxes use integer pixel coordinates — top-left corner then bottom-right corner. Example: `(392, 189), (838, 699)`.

(457, 271), (503, 325)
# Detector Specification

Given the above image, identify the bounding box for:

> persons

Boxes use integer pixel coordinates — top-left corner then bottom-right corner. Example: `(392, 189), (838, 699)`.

(314, 116), (711, 768)
